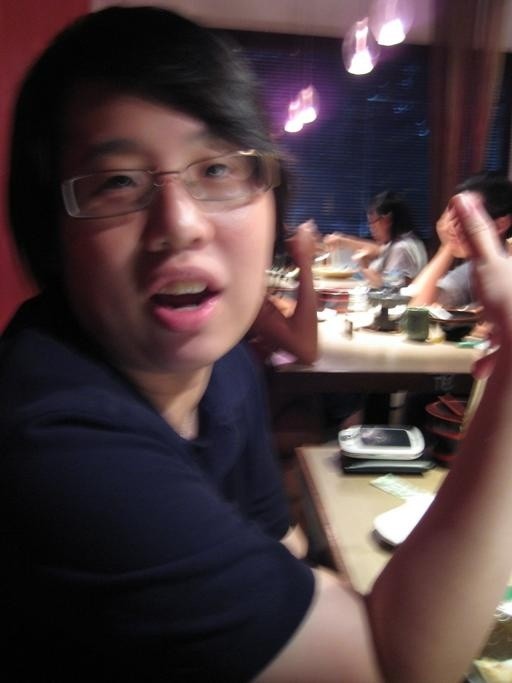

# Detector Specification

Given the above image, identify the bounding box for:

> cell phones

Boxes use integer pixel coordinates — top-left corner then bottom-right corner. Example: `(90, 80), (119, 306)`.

(338, 427), (425, 460)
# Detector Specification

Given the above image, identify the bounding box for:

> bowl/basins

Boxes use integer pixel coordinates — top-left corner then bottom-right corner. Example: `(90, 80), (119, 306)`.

(430, 310), (481, 342)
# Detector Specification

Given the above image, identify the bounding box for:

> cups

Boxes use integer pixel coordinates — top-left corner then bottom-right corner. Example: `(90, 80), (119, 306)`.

(406, 307), (429, 340)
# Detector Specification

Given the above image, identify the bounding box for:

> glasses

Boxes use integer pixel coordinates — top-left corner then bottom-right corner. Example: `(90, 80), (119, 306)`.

(58, 150), (286, 221)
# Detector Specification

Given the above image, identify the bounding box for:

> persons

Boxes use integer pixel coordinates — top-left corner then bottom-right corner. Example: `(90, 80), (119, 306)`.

(1, 6), (511, 683)
(324, 189), (428, 288)
(397, 172), (511, 311)
(253, 217), (318, 366)
